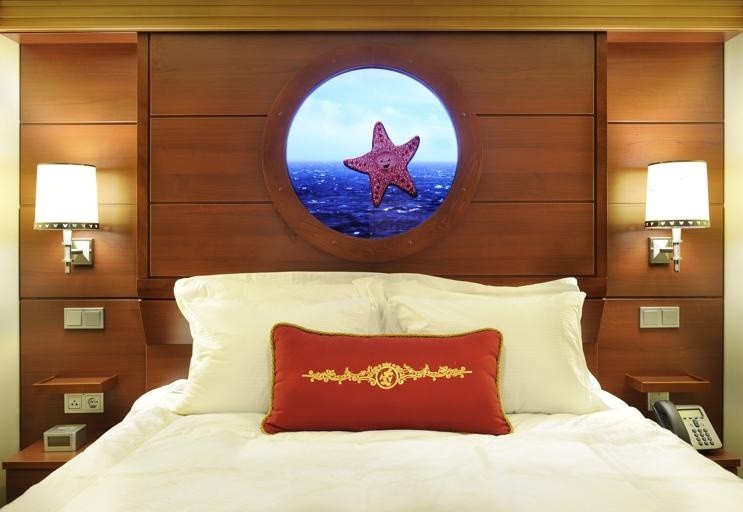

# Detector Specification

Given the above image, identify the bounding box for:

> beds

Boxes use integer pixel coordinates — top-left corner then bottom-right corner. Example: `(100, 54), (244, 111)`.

(1, 380), (743, 512)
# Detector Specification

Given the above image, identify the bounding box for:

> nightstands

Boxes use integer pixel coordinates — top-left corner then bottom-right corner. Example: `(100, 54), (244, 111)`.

(2, 436), (100, 503)
(696, 448), (739, 473)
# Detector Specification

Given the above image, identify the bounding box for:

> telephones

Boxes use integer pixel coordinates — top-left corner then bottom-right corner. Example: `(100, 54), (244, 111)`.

(652, 400), (723, 451)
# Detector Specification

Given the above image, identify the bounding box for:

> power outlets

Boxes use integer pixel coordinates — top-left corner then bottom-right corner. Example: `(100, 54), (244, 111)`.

(63, 392), (104, 414)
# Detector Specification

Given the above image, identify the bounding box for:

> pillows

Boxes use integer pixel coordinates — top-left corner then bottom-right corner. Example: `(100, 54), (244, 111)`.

(258, 319), (514, 436)
(380, 282), (606, 415)
(172, 279), (355, 300)
(176, 299), (381, 416)
(361, 272), (585, 303)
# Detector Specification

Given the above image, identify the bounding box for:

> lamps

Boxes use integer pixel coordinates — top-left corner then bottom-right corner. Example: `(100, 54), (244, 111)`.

(642, 159), (712, 271)
(33, 163), (100, 274)
